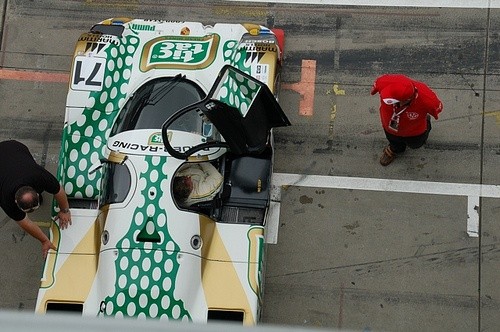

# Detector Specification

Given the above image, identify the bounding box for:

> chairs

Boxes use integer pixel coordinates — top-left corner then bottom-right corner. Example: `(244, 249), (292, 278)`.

(190, 158), (225, 214)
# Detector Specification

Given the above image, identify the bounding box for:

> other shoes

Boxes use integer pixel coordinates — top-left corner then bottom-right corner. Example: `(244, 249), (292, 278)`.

(380, 146), (397, 166)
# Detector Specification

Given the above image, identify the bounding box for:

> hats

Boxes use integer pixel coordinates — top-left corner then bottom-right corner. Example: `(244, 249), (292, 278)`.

(381, 79), (414, 105)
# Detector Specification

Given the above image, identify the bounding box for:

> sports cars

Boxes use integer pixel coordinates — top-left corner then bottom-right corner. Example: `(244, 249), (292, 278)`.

(33, 17), (293, 324)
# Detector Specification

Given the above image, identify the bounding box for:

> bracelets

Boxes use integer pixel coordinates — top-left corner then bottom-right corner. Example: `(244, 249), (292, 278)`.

(60, 208), (69, 213)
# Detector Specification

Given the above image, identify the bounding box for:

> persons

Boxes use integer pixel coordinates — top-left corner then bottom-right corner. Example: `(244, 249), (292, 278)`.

(0, 140), (72, 260)
(172, 162), (224, 211)
(371, 74), (443, 166)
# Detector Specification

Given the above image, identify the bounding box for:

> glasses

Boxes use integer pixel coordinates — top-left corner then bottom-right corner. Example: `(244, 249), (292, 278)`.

(18, 201), (40, 212)
